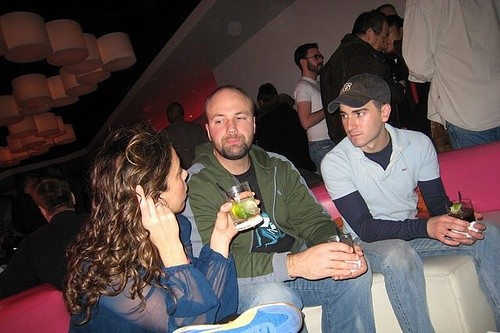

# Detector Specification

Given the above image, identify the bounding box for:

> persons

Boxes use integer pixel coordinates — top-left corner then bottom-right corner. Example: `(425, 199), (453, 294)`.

(0, 173), (89, 301)
(181, 85), (376, 333)
(376, 3), (452, 153)
(293, 43), (336, 170)
(252, 82), (316, 173)
(320, 73), (500, 333)
(402, 0), (500, 151)
(64, 120), (303, 333)
(160, 102), (209, 171)
(320, 10), (430, 144)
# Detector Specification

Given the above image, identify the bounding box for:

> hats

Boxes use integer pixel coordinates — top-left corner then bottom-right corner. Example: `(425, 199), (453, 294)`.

(327, 74), (391, 113)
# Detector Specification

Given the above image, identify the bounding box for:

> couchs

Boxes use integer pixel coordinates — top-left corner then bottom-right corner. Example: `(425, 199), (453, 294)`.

(0, 138), (500, 333)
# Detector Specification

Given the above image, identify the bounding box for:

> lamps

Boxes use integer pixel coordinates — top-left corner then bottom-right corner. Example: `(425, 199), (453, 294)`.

(0, 12), (136, 168)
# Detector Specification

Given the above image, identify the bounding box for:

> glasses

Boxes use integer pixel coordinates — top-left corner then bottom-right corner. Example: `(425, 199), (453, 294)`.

(304, 54), (324, 60)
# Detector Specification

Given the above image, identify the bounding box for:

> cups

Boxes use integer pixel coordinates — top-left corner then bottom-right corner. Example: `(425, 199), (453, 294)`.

(327, 233), (355, 254)
(446, 198), (475, 223)
(220, 181), (263, 231)
